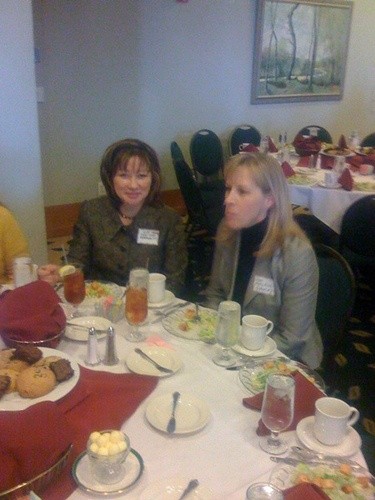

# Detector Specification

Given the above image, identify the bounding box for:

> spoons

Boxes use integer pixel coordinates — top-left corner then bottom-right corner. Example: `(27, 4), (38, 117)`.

(135, 346), (173, 373)
(292, 446), (358, 465)
(167, 390), (180, 434)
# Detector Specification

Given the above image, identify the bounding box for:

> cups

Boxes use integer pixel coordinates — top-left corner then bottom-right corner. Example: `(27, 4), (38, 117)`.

(86, 429), (131, 484)
(11, 255), (33, 288)
(129, 268), (149, 280)
(313, 397), (360, 447)
(247, 482), (285, 500)
(147, 274), (168, 303)
(93, 299), (125, 321)
(240, 315), (274, 348)
(33, 264), (39, 283)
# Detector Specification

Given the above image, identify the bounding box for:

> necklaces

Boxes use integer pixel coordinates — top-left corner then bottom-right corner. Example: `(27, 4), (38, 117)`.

(118, 208), (133, 220)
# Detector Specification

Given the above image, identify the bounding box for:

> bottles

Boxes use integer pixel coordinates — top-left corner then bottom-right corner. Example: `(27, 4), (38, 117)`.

(85, 327), (102, 365)
(103, 326), (119, 365)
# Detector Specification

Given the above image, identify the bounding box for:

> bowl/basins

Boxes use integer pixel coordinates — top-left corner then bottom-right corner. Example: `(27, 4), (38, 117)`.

(1, 324), (66, 352)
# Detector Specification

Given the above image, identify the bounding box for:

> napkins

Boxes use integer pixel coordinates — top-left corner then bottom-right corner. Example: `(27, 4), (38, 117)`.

(33, 365), (159, 500)
(244, 370), (328, 435)
(339, 135), (348, 148)
(0, 279), (65, 343)
(0, 400), (69, 492)
(296, 136), (321, 152)
(296, 155), (335, 169)
(350, 156), (362, 171)
(282, 161), (294, 176)
(339, 168), (353, 191)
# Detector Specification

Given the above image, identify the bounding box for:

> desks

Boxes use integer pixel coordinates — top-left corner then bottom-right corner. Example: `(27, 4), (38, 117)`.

(255, 144), (375, 237)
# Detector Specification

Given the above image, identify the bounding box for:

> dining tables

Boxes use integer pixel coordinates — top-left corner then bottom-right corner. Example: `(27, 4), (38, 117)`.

(0, 274), (375, 500)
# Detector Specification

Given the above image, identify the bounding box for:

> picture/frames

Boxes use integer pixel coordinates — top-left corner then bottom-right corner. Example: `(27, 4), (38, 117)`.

(250, 0), (355, 106)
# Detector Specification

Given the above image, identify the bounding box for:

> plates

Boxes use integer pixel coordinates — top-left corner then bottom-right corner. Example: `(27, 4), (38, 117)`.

(0, 280), (360, 496)
(241, 138), (375, 192)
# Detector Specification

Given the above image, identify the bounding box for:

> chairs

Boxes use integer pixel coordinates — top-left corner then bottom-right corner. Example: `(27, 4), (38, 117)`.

(171, 124), (375, 381)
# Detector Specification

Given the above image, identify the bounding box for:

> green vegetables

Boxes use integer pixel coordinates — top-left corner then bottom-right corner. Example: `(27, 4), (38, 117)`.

(353, 181), (375, 192)
(187, 311), (219, 340)
(252, 357), (288, 390)
(291, 463), (375, 500)
(288, 174), (316, 186)
(85, 283), (114, 298)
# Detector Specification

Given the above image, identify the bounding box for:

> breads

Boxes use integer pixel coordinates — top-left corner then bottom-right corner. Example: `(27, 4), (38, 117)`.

(0, 343), (74, 400)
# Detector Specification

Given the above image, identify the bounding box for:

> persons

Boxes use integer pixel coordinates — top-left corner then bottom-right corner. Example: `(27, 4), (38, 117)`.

(204, 153), (323, 371)
(0, 206), (30, 284)
(37, 138), (190, 296)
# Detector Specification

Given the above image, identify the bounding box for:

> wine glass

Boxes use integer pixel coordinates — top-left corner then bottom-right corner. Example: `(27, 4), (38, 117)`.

(214, 299), (241, 367)
(125, 281), (147, 342)
(63, 263), (85, 319)
(260, 373), (293, 455)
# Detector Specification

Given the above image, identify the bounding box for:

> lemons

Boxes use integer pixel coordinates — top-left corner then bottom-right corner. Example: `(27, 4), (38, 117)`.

(59, 265), (76, 276)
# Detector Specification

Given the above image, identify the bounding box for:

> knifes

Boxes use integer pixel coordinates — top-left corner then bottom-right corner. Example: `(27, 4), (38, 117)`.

(270, 457), (360, 472)
